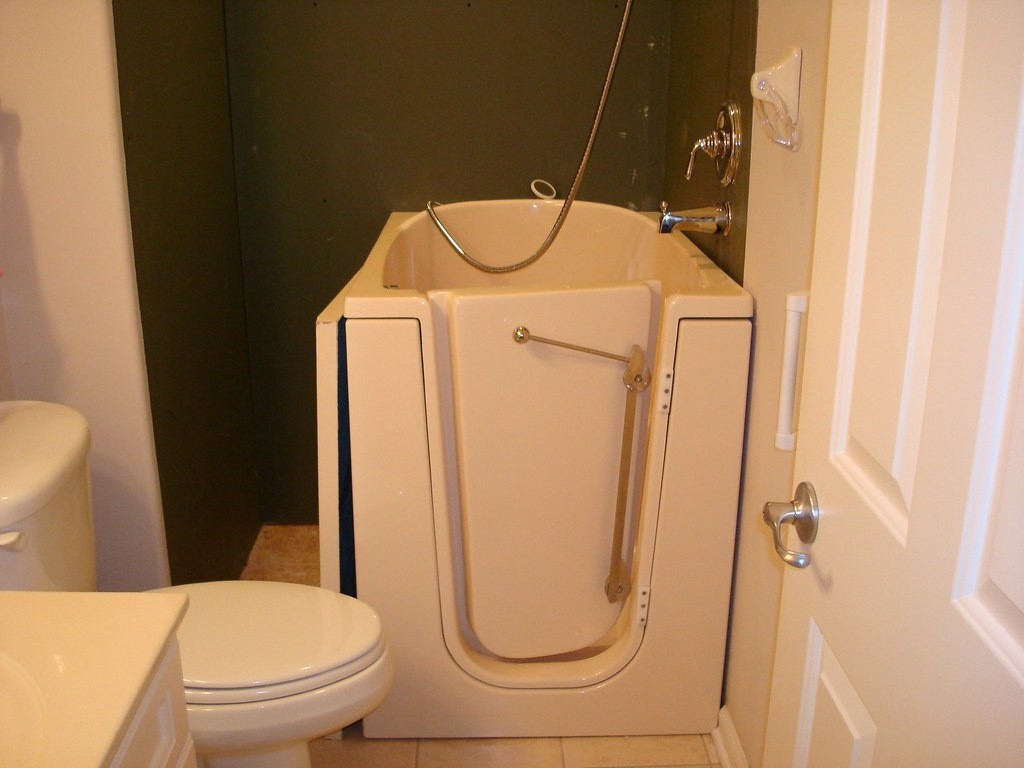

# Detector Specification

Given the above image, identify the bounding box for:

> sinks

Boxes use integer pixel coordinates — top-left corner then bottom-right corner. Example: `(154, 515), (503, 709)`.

(381, 198), (711, 298)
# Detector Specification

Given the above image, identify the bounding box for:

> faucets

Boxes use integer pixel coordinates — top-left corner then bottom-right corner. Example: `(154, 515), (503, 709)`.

(659, 201), (733, 237)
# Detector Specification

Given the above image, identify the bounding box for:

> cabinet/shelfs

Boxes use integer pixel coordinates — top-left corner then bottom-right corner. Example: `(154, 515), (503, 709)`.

(110, 633), (197, 768)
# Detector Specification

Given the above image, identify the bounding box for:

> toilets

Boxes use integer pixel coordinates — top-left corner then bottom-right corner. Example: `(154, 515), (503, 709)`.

(0, 396), (397, 768)
